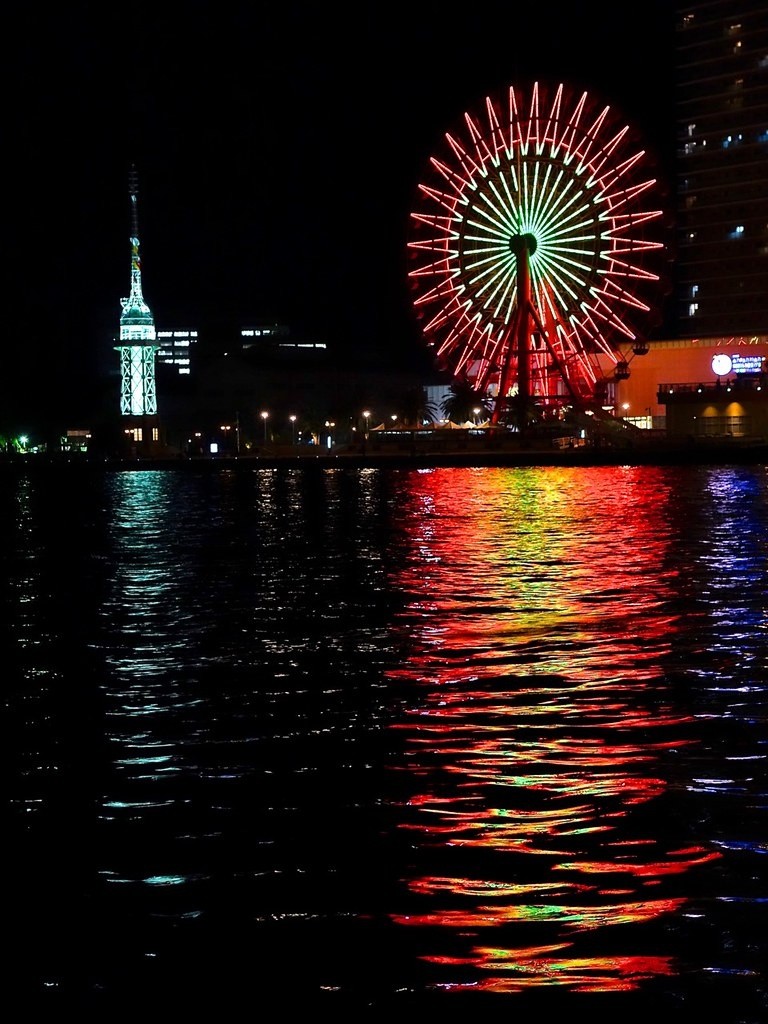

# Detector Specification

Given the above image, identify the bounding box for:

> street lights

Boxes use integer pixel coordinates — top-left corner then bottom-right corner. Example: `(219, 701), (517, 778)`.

(259, 409), (398, 446)
